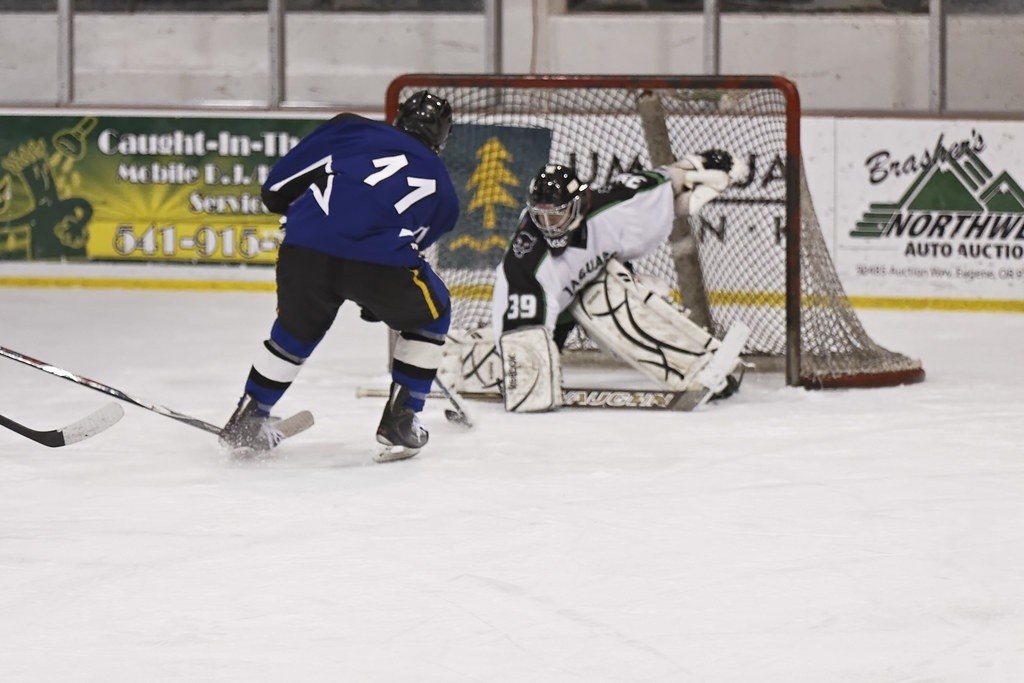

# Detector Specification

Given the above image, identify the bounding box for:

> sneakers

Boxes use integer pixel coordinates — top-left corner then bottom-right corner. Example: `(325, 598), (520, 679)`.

(376, 383), (429, 447)
(219, 394), (280, 451)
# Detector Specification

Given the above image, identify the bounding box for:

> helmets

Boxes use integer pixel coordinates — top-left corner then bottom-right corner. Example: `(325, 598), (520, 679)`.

(527, 165), (589, 238)
(395, 90), (452, 154)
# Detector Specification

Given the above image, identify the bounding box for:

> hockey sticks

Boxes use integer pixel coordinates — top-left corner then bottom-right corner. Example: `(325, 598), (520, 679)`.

(279, 216), (473, 428)
(0, 402), (124, 447)
(355, 319), (752, 412)
(0, 346), (314, 454)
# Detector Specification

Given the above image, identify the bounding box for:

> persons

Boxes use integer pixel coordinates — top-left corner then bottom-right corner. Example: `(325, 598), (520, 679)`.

(493, 147), (738, 399)
(219, 90), (460, 463)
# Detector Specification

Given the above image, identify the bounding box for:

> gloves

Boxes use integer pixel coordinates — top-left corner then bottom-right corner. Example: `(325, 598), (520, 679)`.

(700, 149), (732, 173)
